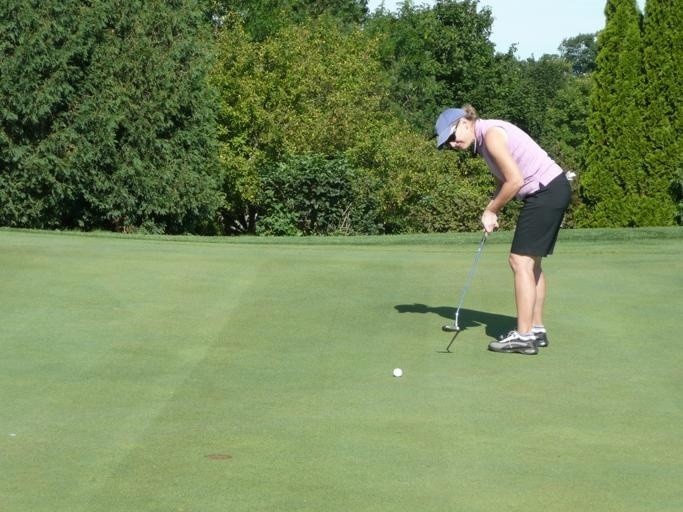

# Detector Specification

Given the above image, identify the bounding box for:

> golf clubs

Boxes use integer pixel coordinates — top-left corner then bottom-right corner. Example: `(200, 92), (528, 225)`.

(443, 231), (487, 333)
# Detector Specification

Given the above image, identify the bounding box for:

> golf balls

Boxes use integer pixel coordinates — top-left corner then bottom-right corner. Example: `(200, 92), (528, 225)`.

(394, 368), (401, 377)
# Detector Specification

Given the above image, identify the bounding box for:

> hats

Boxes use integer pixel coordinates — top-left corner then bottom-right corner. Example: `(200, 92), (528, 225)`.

(435, 109), (466, 150)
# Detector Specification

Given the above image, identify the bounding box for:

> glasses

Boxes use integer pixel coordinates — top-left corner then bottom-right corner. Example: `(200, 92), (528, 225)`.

(446, 120), (460, 145)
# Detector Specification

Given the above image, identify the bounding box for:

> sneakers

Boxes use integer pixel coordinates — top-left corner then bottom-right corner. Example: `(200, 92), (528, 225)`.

(488, 328), (548, 354)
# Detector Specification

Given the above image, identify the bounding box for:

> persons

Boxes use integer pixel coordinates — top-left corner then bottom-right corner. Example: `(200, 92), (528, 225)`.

(434, 102), (572, 355)
